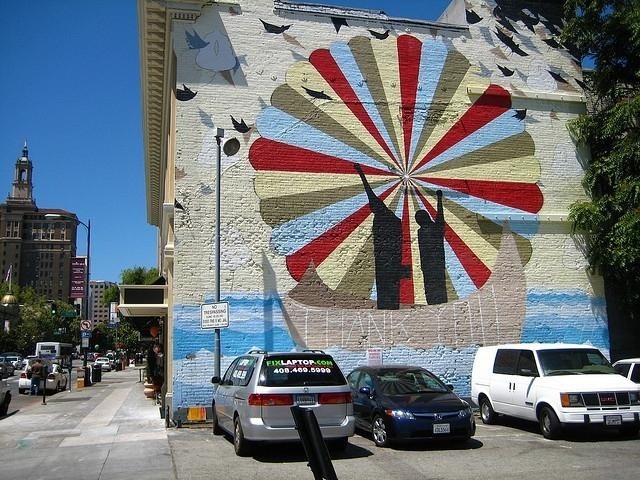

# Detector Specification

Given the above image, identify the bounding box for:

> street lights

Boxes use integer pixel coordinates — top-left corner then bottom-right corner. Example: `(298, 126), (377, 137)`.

(210, 122), (229, 383)
(42, 212), (92, 387)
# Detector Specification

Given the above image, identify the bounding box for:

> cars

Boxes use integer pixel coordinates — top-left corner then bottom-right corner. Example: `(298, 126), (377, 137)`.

(610, 357), (640, 384)
(341, 363), (479, 449)
(0, 348), (128, 416)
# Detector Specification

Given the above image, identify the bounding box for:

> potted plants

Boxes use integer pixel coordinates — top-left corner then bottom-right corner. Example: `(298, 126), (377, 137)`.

(144, 344), (159, 398)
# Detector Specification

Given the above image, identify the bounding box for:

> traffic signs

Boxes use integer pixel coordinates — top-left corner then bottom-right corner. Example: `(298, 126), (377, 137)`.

(199, 301), (229, 330)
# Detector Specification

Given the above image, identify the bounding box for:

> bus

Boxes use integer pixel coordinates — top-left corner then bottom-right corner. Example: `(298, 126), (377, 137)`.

(36, 341), (74, 370)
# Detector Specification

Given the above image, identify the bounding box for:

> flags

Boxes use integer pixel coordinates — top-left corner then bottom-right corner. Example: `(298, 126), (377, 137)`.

(6, 269), (10, 282)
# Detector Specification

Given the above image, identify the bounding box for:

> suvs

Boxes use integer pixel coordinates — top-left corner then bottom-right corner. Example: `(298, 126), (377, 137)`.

(205, 344), (361, 460)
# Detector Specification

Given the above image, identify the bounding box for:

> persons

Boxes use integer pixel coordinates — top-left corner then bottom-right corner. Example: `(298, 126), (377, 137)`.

(28, 359), (44, 396)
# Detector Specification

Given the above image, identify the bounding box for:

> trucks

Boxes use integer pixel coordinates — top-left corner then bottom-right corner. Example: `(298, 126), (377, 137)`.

(469, 340), (640, 442)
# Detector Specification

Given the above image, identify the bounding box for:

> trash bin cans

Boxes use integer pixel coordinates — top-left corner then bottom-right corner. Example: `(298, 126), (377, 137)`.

(91, 363), (101, 382)
(115, 358), (122, 371)
